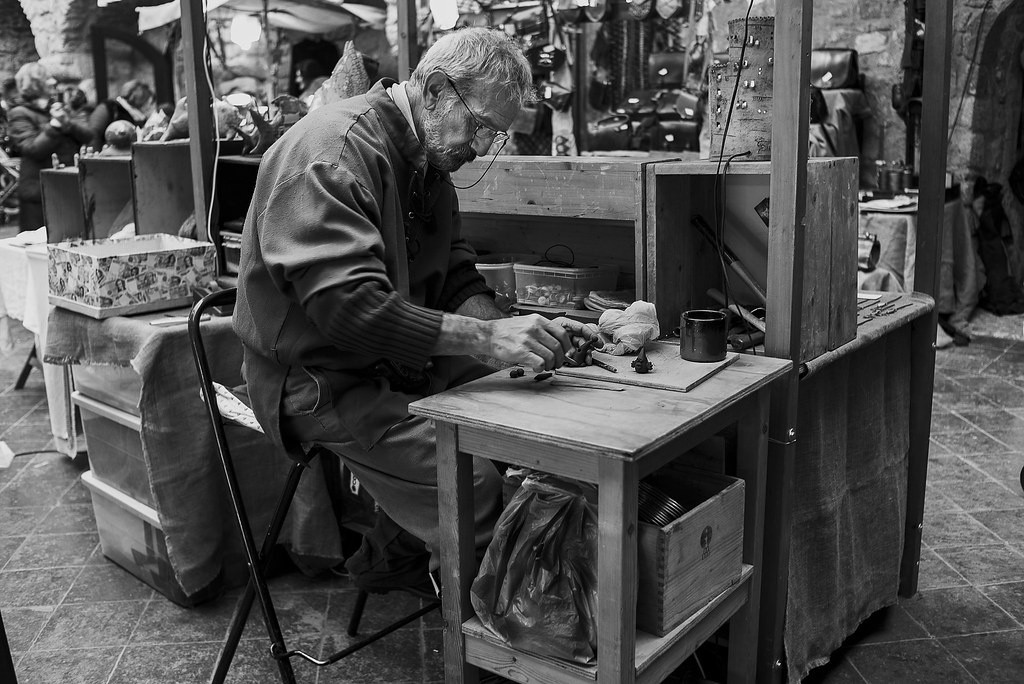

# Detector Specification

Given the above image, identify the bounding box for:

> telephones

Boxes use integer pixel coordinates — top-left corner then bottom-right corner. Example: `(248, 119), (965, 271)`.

(193, 280), (236, 317)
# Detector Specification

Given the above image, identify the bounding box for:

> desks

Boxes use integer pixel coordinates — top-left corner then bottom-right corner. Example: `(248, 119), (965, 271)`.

(856, 200), (986, 347)
(0, 234), (48, 390)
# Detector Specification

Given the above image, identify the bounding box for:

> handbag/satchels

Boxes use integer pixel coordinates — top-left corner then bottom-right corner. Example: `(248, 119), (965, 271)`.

(536, 14), (704, 152)
(811, 49), (859, 90)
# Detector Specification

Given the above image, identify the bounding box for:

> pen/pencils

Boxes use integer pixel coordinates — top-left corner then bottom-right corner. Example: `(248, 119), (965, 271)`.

(592, 357), (618, 373)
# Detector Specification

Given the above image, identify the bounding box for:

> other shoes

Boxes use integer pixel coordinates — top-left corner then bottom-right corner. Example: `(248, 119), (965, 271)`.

(348, 567), (438, 601)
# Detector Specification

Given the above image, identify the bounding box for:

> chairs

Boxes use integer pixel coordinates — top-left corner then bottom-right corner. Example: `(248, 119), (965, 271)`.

(191, 288), (440, 684)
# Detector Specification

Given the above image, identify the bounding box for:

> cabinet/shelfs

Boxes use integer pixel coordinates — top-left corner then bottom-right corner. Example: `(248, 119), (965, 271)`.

(409, 333), (795, 684)
(211, 153), (939, 683)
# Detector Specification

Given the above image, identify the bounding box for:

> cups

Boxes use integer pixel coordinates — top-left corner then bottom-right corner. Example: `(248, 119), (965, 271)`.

(680, 310), (729, 362)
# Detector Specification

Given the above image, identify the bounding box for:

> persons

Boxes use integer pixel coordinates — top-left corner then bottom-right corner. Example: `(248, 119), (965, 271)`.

(6, 62), (84, 233)
(64, 88), (93, 119)
(88, 80), (154, 152)
(231, 28), (604, 684)
(294, 59), (327, 99)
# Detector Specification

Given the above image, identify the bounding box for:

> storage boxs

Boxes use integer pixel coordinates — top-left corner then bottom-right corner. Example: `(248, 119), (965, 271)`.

(513, 259), (620, 311)
(501, 463), (745, 637)
(474, 252), (539, 305)
(71, 360), (299, 607)
(46, 232), (216, 320)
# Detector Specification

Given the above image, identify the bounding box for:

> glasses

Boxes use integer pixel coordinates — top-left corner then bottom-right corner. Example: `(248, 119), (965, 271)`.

(438, 67), (510, 144)
